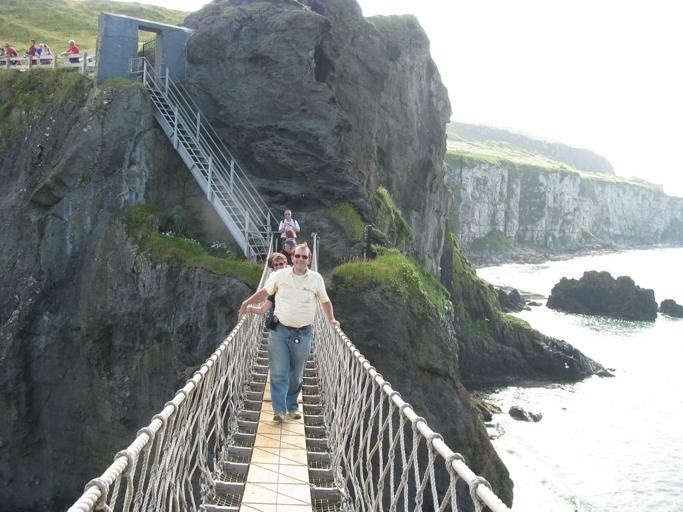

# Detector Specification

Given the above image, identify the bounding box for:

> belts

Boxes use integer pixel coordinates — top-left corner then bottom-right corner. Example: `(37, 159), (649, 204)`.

(284, 324), (312, 330)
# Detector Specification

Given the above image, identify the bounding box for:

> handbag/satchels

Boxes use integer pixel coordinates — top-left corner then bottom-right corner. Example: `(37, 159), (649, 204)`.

(265, 312), (279, 331)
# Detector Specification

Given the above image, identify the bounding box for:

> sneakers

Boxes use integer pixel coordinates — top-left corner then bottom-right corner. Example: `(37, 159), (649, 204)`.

(273, 411), (301, 422)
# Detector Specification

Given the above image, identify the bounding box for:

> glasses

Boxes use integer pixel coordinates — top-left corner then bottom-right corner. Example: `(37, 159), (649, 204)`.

(275, 263), (283, 266)
(285, 213), (290, 215)
(295, 255), (308, 259)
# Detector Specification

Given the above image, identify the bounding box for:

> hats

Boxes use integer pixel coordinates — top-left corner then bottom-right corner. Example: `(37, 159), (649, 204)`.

(283, 230), (297, 247)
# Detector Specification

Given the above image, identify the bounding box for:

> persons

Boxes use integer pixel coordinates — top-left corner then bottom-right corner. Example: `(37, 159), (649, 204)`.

(24, 39), (53, 65)
(61, 40), (80, 74)
(238, 242), (341, 421)
(244, 209), (301, 320)
(0, 42), (22, 68)
